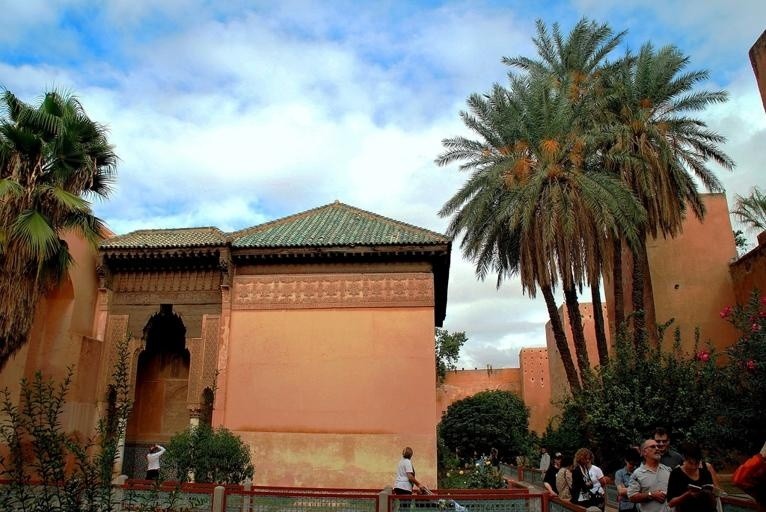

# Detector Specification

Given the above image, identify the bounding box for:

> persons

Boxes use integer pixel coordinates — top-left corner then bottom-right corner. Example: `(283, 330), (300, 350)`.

(393, 445), (421, 512)
(540, 426), (721, 512)
(733, 441), (766, 511)
(489, 447), (502, 470)
(144, 443), (166, 485)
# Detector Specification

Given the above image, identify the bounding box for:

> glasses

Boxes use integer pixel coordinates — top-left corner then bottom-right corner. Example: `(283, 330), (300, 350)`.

(642, 444), (662, 450)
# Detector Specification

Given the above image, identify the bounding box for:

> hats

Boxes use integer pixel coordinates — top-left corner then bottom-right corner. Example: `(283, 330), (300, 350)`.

(555, 452), (564, 458)
